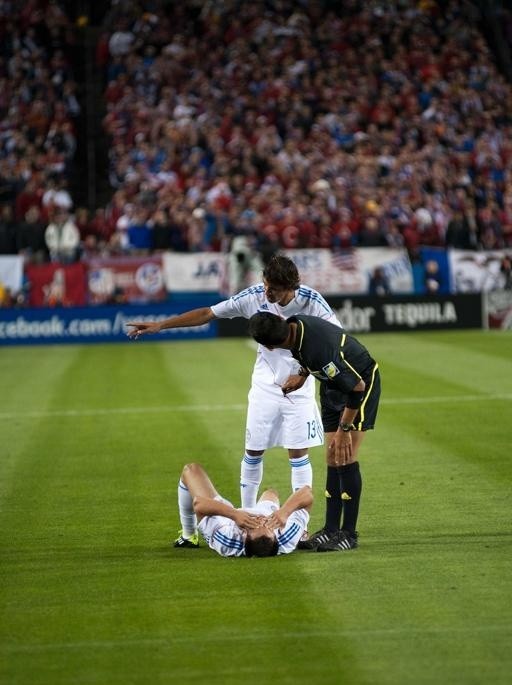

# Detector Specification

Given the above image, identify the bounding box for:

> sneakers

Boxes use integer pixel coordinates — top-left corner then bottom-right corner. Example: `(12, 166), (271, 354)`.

(297, 527), (340, 552)
(172, 532), (202, 550)
(299, 528), (310, 542)
(316, 529), (359, 552)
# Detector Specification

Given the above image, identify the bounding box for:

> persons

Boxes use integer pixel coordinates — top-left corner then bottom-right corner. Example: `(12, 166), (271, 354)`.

(0, 0), (512, 321)
(174, 462), (313, 559)
(249, 311), (382, 552)
(126, 257), (343, 550)
